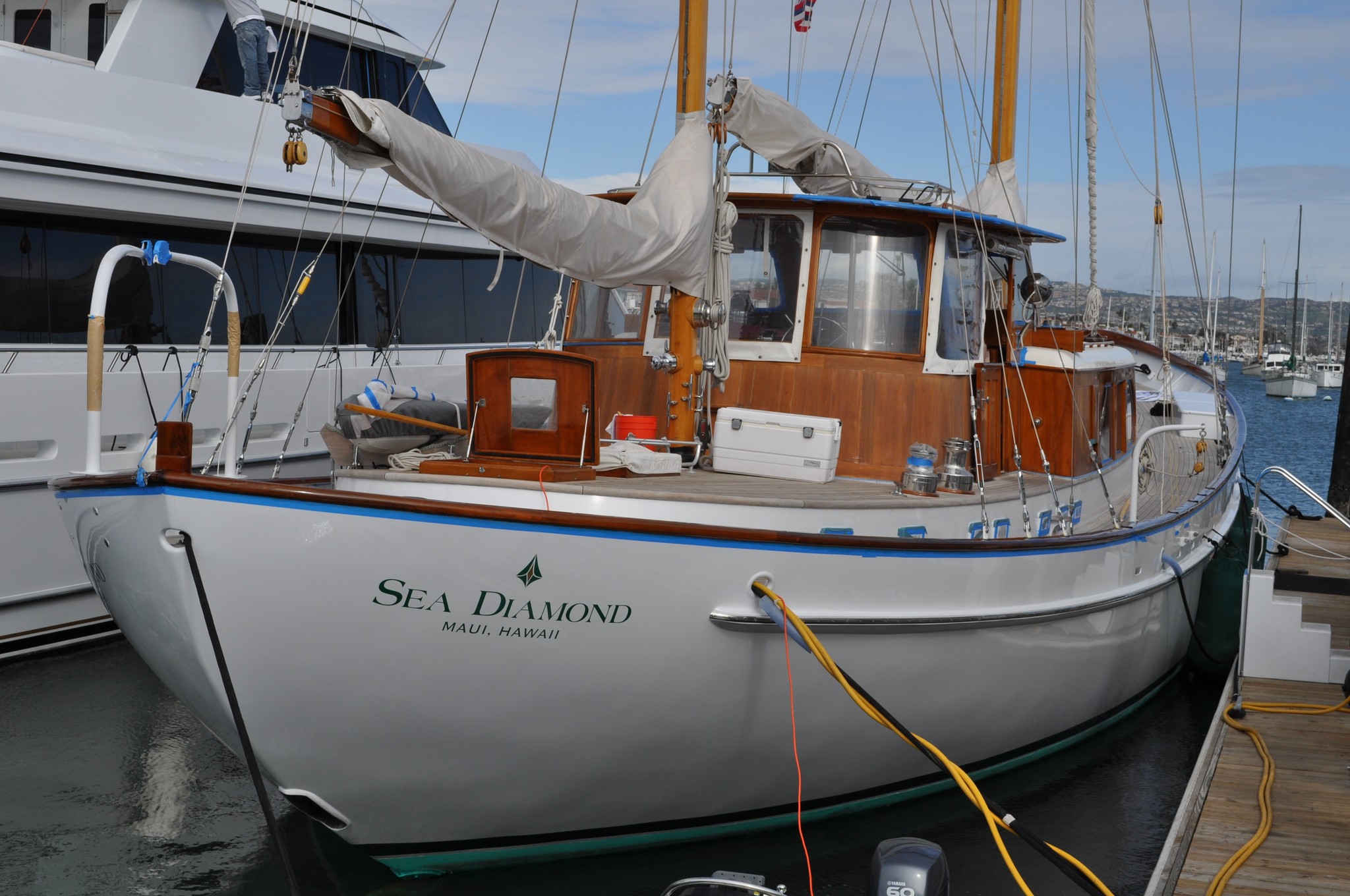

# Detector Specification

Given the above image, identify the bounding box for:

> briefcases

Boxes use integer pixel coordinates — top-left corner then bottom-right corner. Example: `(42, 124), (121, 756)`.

(713, 407), (842, 483)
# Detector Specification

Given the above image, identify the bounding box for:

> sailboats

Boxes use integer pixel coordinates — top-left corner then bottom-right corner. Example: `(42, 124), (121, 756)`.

(40, 0), (1251, 889)
(1149, 195), (1346, 400)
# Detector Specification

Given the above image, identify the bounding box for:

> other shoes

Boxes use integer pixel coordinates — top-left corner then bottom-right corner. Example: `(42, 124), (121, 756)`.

(262, 92), (273, 103)
(239, 94), (261, 101)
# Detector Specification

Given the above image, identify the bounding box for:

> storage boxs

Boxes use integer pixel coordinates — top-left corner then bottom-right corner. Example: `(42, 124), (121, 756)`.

(1032, 326), (1085, 354)
(421, 347), (601, 483)
(711, 403), (843, 485)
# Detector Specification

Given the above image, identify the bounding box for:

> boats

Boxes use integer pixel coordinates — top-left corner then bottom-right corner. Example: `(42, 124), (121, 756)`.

(0, 0), (593, 661)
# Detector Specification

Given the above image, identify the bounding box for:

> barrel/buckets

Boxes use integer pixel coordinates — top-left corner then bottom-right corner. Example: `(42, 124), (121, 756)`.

(615, 415), (658, 452)
